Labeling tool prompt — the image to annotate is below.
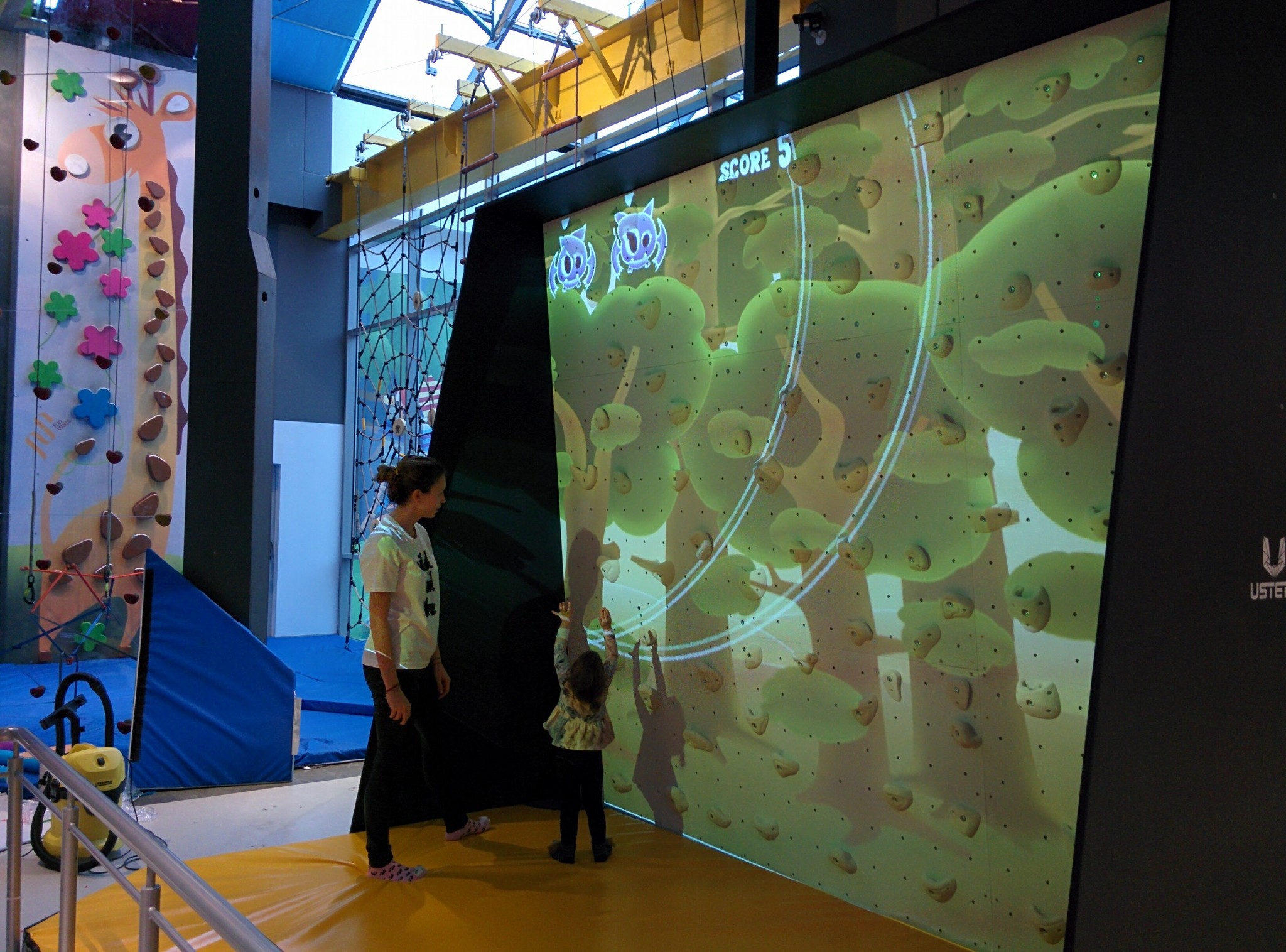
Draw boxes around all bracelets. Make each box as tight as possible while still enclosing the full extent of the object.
[385,682,400,693]
[433,662,442,665]
[432,657,441,662]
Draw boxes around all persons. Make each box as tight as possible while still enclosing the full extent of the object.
[355,454,499,883]
[541,601,620,869]
